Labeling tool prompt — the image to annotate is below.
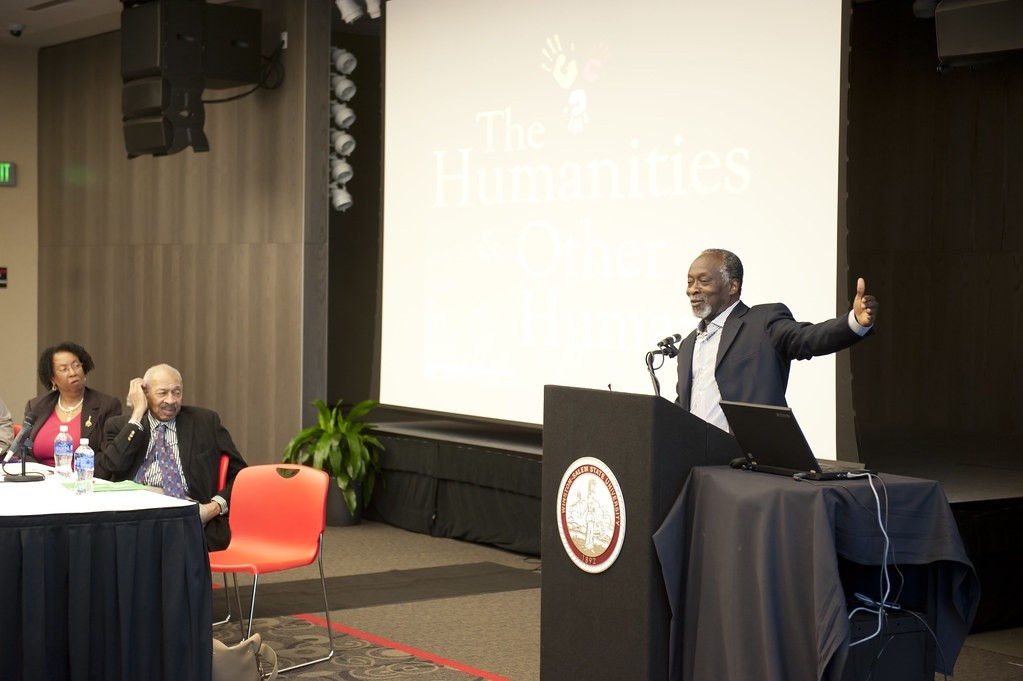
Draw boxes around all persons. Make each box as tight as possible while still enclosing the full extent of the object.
[24,341,122,481]
[675,249,878,437]
[0,401,14,457]
[100,364,246,553]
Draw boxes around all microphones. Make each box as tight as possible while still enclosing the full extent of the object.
[2,412,38,467]
[658,334,681,347]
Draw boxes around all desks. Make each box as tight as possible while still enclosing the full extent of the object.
[0,457,213,681]
[652,460,980,681]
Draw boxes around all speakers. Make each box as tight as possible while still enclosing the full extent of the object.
[935,0,1023,68]
[120,0,264,159]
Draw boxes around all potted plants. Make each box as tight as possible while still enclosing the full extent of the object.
[283,399,385,526]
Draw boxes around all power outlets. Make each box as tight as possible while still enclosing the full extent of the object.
[281,32,287,48]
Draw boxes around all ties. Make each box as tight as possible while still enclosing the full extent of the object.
[134,424,186,499]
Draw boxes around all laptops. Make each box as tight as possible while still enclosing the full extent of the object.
[719,400,878,480]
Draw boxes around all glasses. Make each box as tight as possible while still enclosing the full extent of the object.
[55,363,84,375]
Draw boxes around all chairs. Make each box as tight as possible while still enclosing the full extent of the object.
[208,463,333,677]
[213,453,230,626]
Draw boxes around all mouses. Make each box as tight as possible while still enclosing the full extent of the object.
[729,457,746,468]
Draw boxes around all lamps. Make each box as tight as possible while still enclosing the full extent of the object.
[331,46,357,74]
[330,100,356,128]
[329,183,352,211]
[335,0,363,24]
[365,0,381,19]
[330,73,356,100]
[329,127,355,156]
[329,155,353,183]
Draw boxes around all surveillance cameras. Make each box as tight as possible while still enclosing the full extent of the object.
[9,24,23,37]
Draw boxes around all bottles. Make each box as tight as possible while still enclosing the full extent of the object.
[74,438,95,496]
[54,425,74,483]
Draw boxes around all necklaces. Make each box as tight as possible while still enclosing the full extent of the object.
[58,394,83,413]
